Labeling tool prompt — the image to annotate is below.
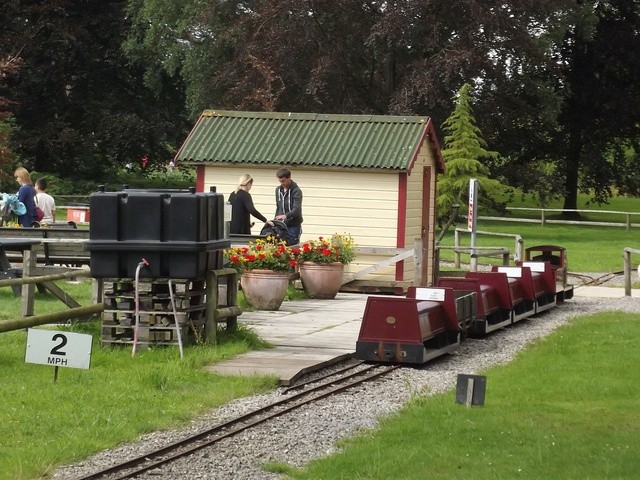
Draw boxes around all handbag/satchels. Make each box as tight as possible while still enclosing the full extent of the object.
[261,218,290,246]
[34,195,44,221]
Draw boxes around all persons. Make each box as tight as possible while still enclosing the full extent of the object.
[274,169,304,247]
[13,166,39,228]
[227,174,274,235]
[33,178,56,224]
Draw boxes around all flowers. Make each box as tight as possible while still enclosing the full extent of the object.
[298,233,360,266]
[229,234,301,273]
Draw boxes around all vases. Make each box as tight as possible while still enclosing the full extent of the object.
[298,260,344,299]
[240,268,288,310]
